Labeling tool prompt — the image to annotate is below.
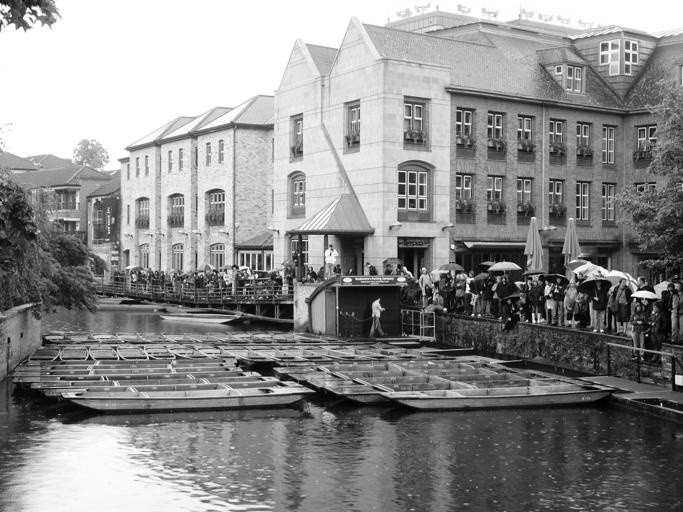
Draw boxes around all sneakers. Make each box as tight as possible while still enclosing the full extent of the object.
[440,307,629,345]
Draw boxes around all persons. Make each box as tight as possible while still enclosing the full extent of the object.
[364,262,378,275]
[367,296,389,339]
[293,249,305,278]
[384,264,395,275]
[111,262,354,301]
[323,244,338,280]
[401,263,682,363]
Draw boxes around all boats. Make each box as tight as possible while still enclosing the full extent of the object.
[91,299,168,312]
[152,308,246,325]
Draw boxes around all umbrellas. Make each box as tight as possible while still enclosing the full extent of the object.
[486,260,522,279]
[523,216,543,282]
[381,257,405,266]
[480,262,494,267]
[565,260,593,270]
[560,216,582,276]
